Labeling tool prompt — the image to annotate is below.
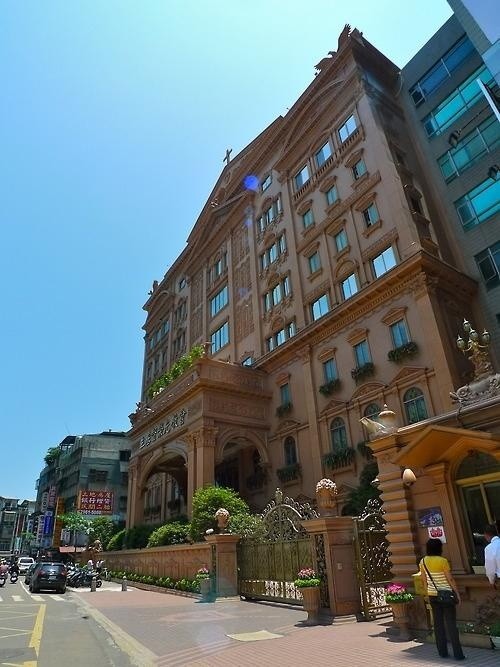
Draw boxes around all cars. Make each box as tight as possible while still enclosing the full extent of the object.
[23,563,34,584]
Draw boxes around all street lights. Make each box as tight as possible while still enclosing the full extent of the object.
[455,317,497,384]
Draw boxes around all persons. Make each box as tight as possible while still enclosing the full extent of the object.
[483,525,500,592]
[0,561,20,575]
[418,539,465,660]
[87,558,106,571]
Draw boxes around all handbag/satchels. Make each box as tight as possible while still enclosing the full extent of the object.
[437,590,457,605]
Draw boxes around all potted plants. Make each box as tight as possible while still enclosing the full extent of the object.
[490,623,500,649]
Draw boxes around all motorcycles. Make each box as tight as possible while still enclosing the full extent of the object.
[0,562,19,588]
[66,564,104,590]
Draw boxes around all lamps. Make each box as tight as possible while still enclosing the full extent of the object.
[402,467,417,487]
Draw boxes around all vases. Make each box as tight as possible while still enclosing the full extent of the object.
[198,577,210,602]
[297,585,320,626]
[388,600,414,640]
[460,633,493,649]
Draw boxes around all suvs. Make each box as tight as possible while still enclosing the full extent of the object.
[24,563,67,594]
[15,556,36,575]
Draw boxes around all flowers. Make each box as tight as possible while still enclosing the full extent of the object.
[293,567,320,587]
[196,567,210,578]
[384,584,414,603]
[463,622,490,634]
